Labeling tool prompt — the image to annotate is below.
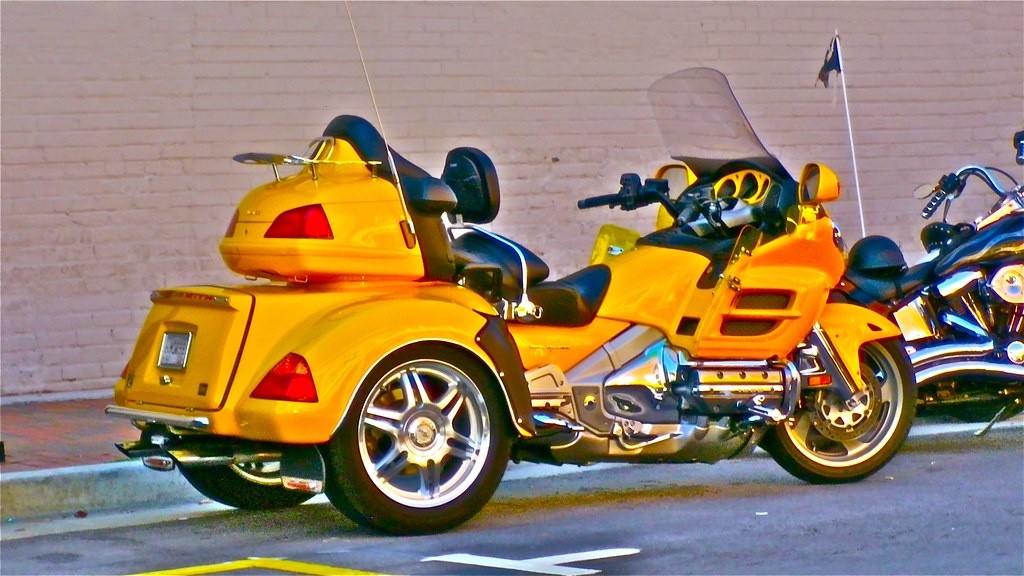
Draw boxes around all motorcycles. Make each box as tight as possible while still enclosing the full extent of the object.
[876,130,1024,438]
[98,68,916,539]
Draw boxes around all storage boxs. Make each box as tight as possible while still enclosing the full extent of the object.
[218,139,423,284]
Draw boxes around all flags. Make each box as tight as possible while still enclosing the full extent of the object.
[814,35,841,89]
